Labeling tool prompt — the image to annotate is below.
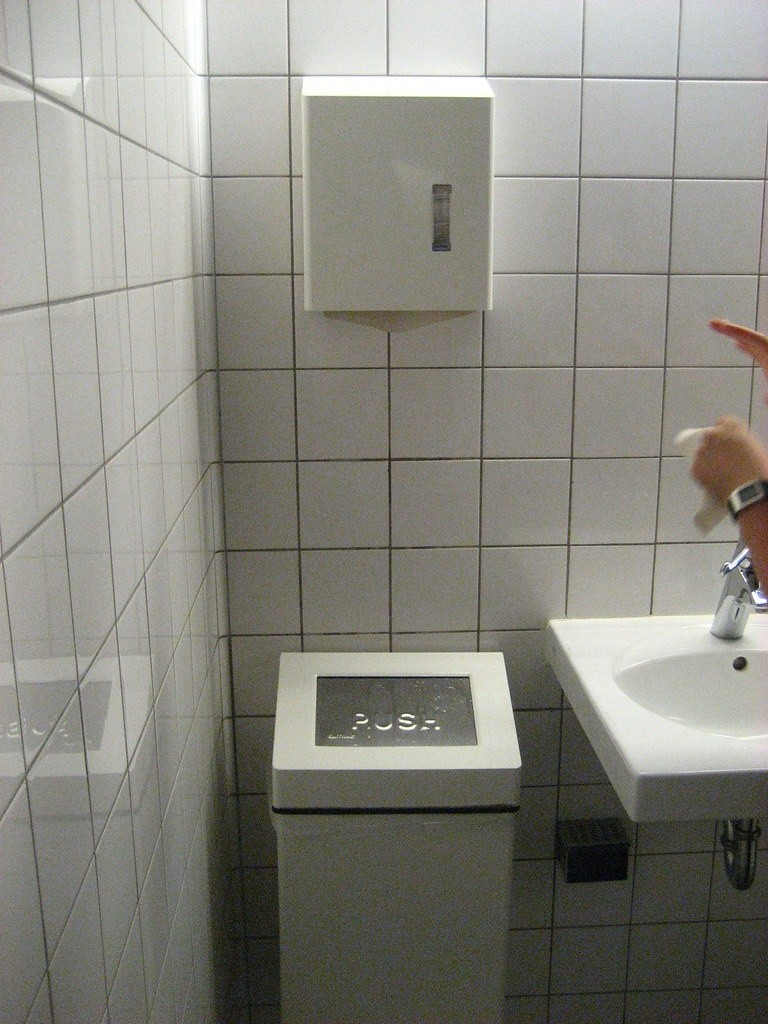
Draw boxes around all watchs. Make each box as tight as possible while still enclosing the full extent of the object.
[728,479,768,525]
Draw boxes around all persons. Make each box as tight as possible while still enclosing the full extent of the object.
[690,321,768,594]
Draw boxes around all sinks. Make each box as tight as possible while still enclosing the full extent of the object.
[542,609,768,823]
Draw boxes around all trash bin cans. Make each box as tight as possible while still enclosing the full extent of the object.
[267,651,524,1024]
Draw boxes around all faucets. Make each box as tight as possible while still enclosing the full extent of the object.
[708,535,768,641]
